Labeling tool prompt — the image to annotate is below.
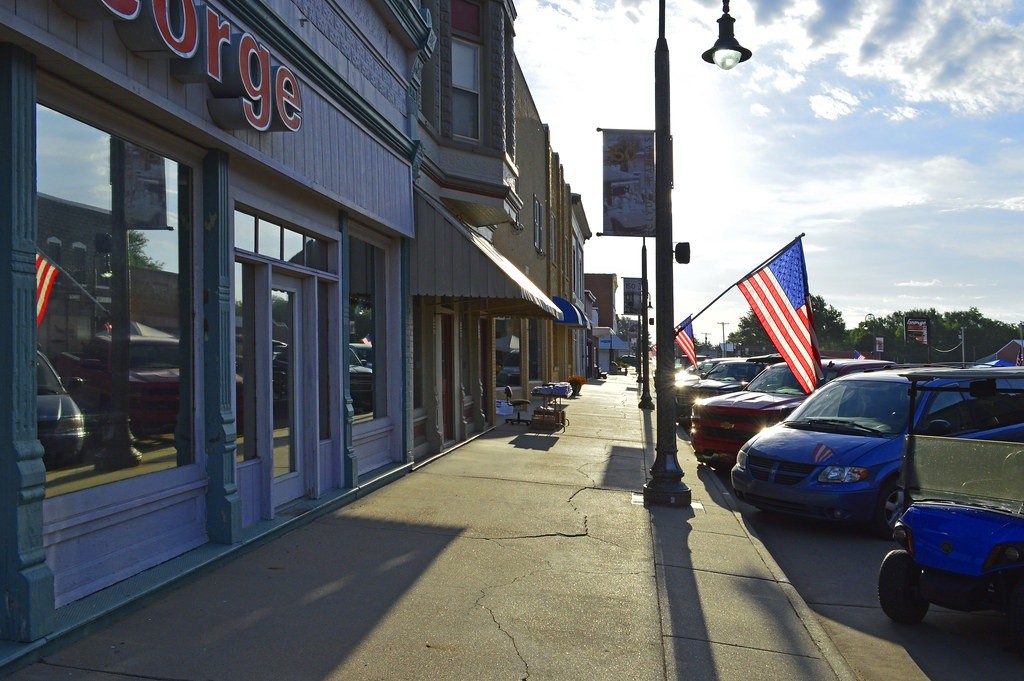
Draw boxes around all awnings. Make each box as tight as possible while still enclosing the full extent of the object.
[410,186,564,321]
[553,295,591,329]
[613,335,633,350]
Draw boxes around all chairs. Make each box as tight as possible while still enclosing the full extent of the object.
[504,385,531,425]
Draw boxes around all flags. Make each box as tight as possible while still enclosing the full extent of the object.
[35,253,60,328]
[736,235,824,394]
[853,349,866,359]
[813,442,834,463]
[1016,347,1022,366]
[674,314,698,369]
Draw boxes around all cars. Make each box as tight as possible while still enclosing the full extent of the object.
[878,363,1024,655]
[675,352,784,424]
[730,364,1024,540]
[689,357,911,468]
[498,348,538,381]
[93,332,246,437]
[35,343,92,468]
[237,334,372,408]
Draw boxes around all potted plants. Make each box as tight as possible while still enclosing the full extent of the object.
[567,375,587,396]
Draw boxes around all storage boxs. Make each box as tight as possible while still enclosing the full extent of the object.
[532,410,565,430]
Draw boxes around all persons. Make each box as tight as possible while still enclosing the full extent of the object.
[83,316,112,443]
[496,358,505,374]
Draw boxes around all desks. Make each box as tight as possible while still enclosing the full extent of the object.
[530,390,573,432]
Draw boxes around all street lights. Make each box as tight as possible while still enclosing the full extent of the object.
[634,290,654,384]
[861,313,877,360]
[700,332,712,355]
[717,322,729,358]
[643,0,753,509]
